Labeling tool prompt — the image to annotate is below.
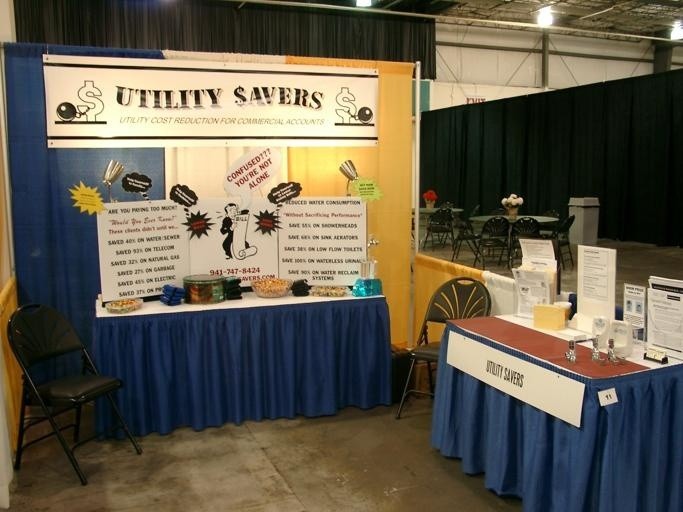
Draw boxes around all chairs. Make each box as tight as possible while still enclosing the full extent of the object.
[5,302,143,485]
[423,201,575,275]
[394,276,492,418]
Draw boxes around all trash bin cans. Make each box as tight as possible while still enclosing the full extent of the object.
[568,197,600,260]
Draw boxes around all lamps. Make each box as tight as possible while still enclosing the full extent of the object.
[338,159,358,195]
[103,160,125,204]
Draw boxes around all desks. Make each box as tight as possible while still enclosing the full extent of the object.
[433,312,676,512]
[95,288,390,438]
[419,207,465,243]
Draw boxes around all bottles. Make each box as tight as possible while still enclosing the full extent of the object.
[566,337,619,367]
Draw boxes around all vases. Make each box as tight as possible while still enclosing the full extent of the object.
[425,199,435,209]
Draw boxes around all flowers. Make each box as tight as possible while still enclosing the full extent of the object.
[422,190,437,200]
[502,193,524,208]
